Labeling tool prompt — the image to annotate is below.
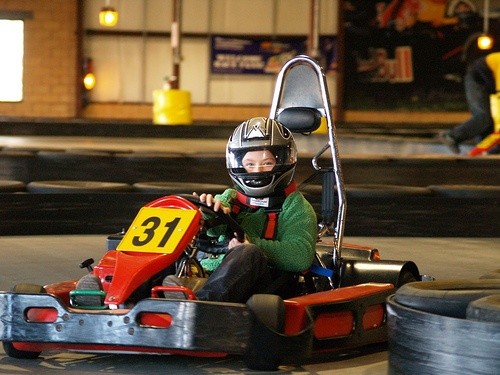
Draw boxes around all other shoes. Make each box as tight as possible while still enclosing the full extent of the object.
[76,275,103,306]
[162,275,187,300]
[433,129,462,153]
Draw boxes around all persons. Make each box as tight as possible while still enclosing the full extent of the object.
[440,52,500,154]
[73,116,319,302]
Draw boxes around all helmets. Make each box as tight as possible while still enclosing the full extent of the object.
[226,117,298,198]
